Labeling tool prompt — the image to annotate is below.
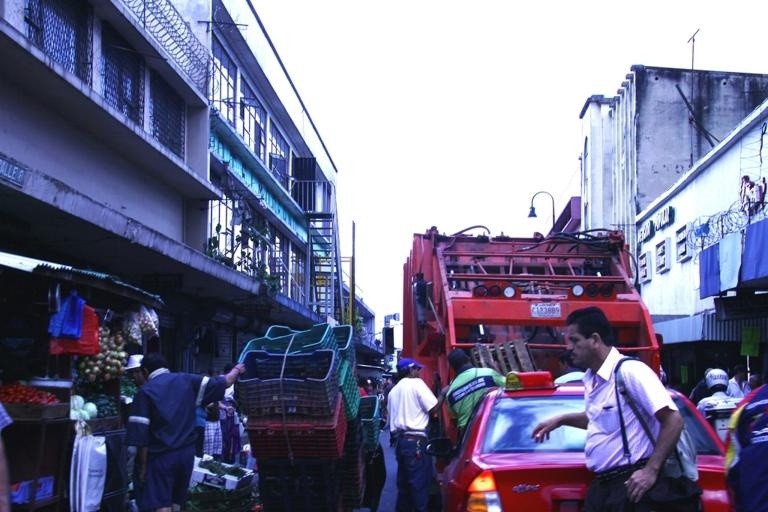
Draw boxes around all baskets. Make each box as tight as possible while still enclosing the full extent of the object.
[3,403,70,418]
[233,323,381,512]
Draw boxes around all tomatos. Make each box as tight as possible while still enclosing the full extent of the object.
[0,383,60,405]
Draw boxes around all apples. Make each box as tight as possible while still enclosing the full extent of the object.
[78,313,160,390]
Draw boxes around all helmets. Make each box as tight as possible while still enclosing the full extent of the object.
[706,369,728,389]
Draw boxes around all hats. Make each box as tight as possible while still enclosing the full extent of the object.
[448,348,464,362]
[396,358,425,371]
[123,354,145,370]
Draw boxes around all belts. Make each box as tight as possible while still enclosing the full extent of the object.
[594,458,649,480]
[398,434,422,440]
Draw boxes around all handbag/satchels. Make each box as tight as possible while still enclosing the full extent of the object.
[628,475,704,510]
[70,421,107,512]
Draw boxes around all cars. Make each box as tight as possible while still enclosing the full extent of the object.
[426,367,737,511]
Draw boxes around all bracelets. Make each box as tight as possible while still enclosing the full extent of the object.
[440,392,446,397]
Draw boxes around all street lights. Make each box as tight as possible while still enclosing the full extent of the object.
[528,191,557,235]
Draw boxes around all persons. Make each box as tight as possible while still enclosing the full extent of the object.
[121,394,134,482]
[354,371,399,447]
[554,351,586,384]
[529,305,684,511]
[121,353,147,387]
[722,382,767,512]
[388,358,454,512]
[124,350,246,512]
[193,362,245,462]
[0,402,14,512]
[446,348,506,441]
[687,365,761,417]
